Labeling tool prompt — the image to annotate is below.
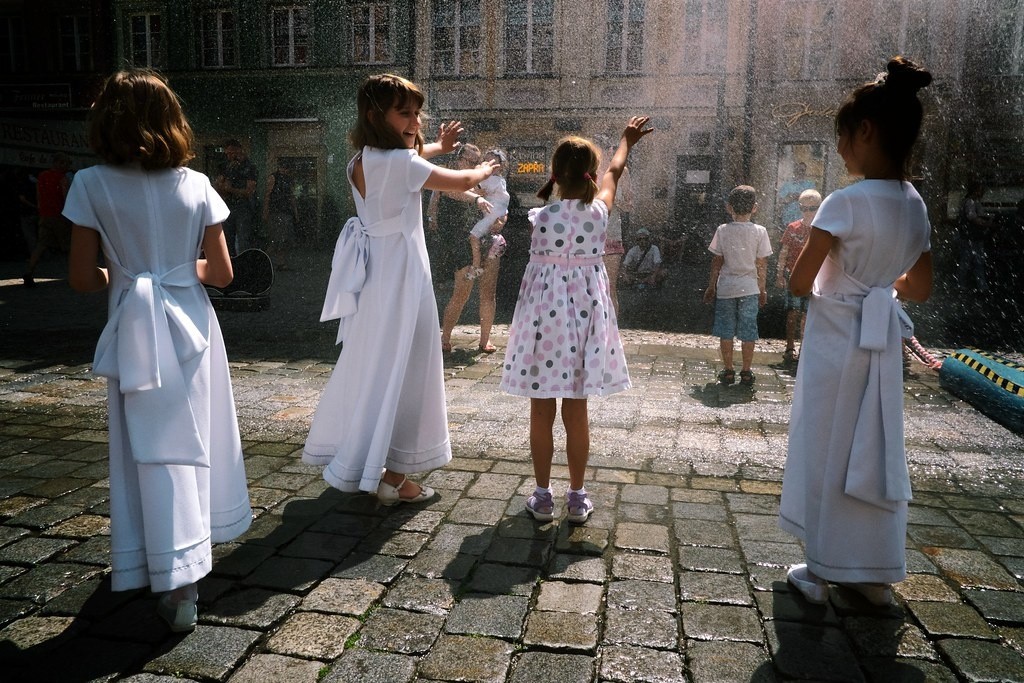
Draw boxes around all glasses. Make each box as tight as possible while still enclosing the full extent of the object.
[635,236,649,242]
[798,202,819,212]
[459,156,478,166]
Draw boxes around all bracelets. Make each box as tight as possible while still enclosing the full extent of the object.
[475,195,483,207]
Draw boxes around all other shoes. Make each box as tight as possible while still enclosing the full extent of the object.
[852,579,892,605]
[789,564,830,603]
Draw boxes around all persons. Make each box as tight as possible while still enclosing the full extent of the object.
[954,182,996,318]
[776,189,822,365]
[499,115,654,521]
[214,142,300,270]
[428,142,510,352]
[63,68,250,633]
[591,134,633,317]
[781,58,934,605]
[705,186,773,384]
[620,229,668,292]
[778,162,814,226]
[301,74,501,504]
[22,154,69,285]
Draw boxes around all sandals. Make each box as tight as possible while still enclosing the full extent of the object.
[526,493,555,520]
[441,342,452,352]
[157,597,198,632]
[376,476,435,507]
[716,368,736,384]
[463,265,487,283]
[567,492,594,523]
[739,369,756,386]
[782,349,799,362]
[480,340,497,352]
